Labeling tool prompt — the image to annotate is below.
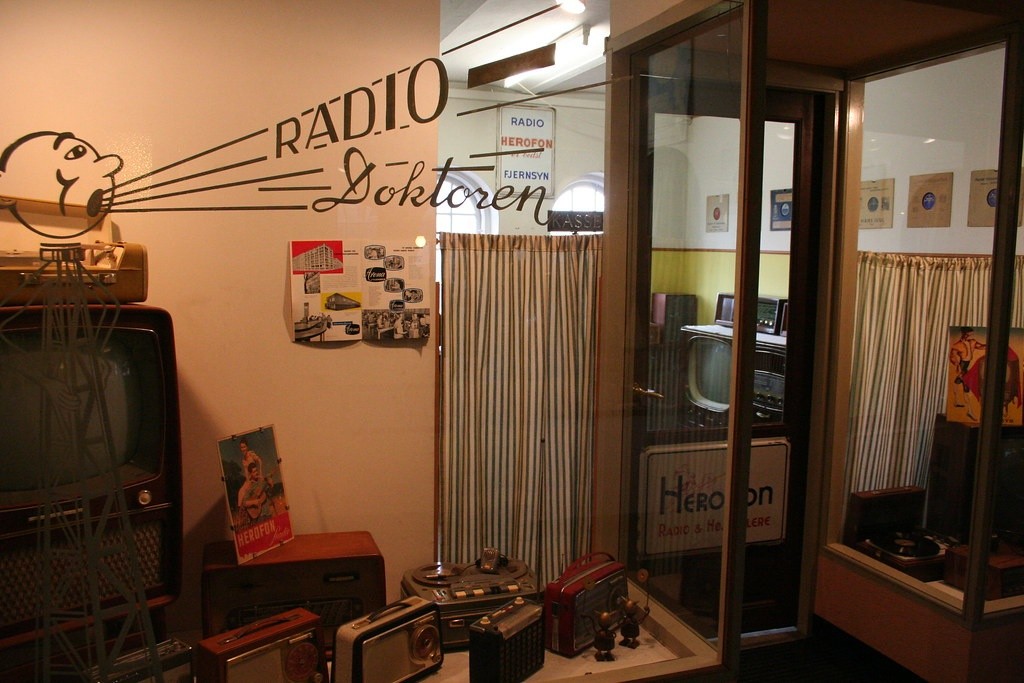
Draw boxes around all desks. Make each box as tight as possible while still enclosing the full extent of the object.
[816,542,1024,683]
[326,578,722,683]
[649,347,685,430]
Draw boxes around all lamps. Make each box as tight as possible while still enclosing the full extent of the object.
[556,0,586,14]
[502,23,590,87]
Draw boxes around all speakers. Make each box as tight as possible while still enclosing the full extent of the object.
[0,503,180,639]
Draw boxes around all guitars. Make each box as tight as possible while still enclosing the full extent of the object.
[244,472,275,521]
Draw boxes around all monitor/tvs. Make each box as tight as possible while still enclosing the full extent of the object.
[714,291,788,334]
[0,305,182,535]
[680,323,787,427]
[927,414,1024,547]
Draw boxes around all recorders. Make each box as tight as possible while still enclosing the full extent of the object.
[544,552,630,658]
[468,596,545,683]
[330,597,444,683]
[83,637,194,683]
[201,530,386,662]
[196,606,329,683]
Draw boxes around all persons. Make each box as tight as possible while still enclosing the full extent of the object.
[363,311,429,337]
[391,301,395,309]
[238,441,274,524]
[386,280,401,291]
[367,269,385,280]
[367,247,382,258]
[385,257,402,268]
[405,290,421,301]
